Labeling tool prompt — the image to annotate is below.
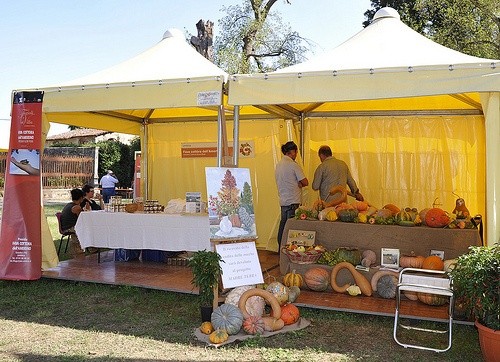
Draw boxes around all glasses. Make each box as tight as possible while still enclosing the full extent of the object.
[89,190,94,193]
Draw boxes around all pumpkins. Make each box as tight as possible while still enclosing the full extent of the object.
[281,184,471,306]
[200,281,299,344]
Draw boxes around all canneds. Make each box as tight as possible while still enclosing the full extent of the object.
[107,196,127,212]
[143,200,162,211]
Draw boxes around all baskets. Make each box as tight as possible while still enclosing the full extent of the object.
[282,247,322,264]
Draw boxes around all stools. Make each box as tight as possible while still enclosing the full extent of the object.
[393,268,454,353]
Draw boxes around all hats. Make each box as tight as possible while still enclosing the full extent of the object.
[108,170,113,174]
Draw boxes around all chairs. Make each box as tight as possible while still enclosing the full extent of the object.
[55,212,76,255]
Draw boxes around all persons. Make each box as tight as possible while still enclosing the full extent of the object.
[312,146,364,203]
[60,185,104,253]
[10,157,39,175]
[100,171,118,203]
[275,141,309,251]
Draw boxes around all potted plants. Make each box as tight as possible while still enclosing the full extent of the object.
[186,249,226,323]
[444,243,500,362]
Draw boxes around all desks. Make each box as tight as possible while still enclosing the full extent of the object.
[91,188,133,201]
[74,210,214,263]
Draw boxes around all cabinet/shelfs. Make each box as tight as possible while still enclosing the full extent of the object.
[280,217,482,295]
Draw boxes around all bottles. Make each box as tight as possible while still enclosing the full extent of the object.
[86,202,90,211]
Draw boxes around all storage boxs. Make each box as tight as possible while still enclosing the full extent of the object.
[113,247,169,264]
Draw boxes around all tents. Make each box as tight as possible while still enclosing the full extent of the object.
[229,8,500,245]
[11,30,300,253]
